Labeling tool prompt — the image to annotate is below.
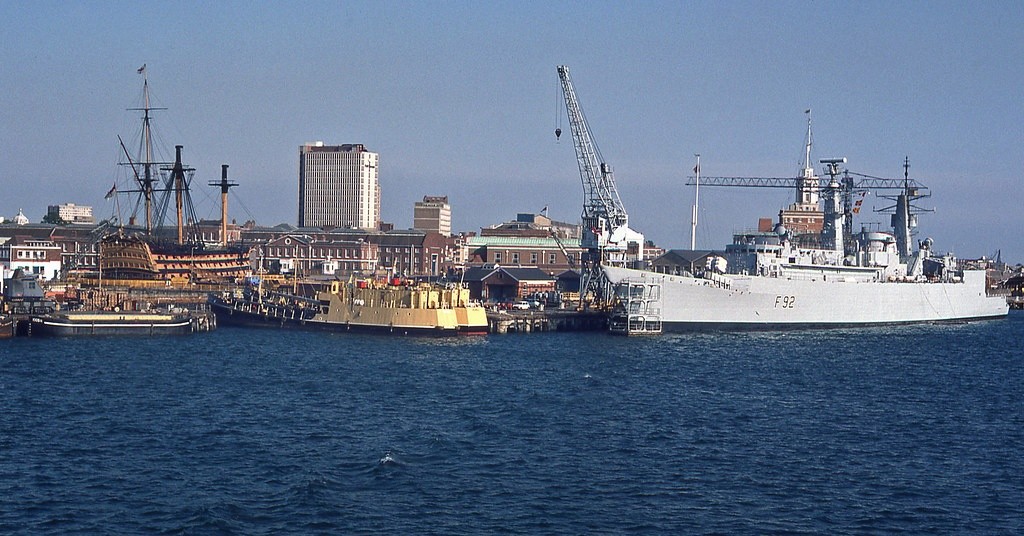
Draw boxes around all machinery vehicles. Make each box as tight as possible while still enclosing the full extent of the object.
[554,64,631,251]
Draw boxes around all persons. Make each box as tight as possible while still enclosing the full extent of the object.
[171,306,189,314]
[56,302,60,311]
[145,301,157,313]
[534,289,548,307]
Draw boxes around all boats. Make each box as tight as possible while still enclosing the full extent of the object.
[211,268,490,337]
[26,308,193,335]
[600,155,1011,334]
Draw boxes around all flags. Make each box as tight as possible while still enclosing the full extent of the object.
[853,192,866,213]
[541,206,547,212]
